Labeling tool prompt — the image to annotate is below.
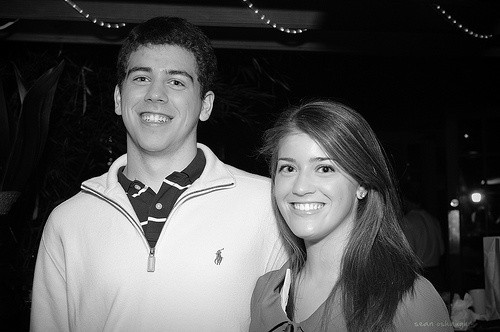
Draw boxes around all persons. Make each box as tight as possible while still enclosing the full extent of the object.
[398,182,446,289]
[248,100,458,332]
[31,16,295,332]
